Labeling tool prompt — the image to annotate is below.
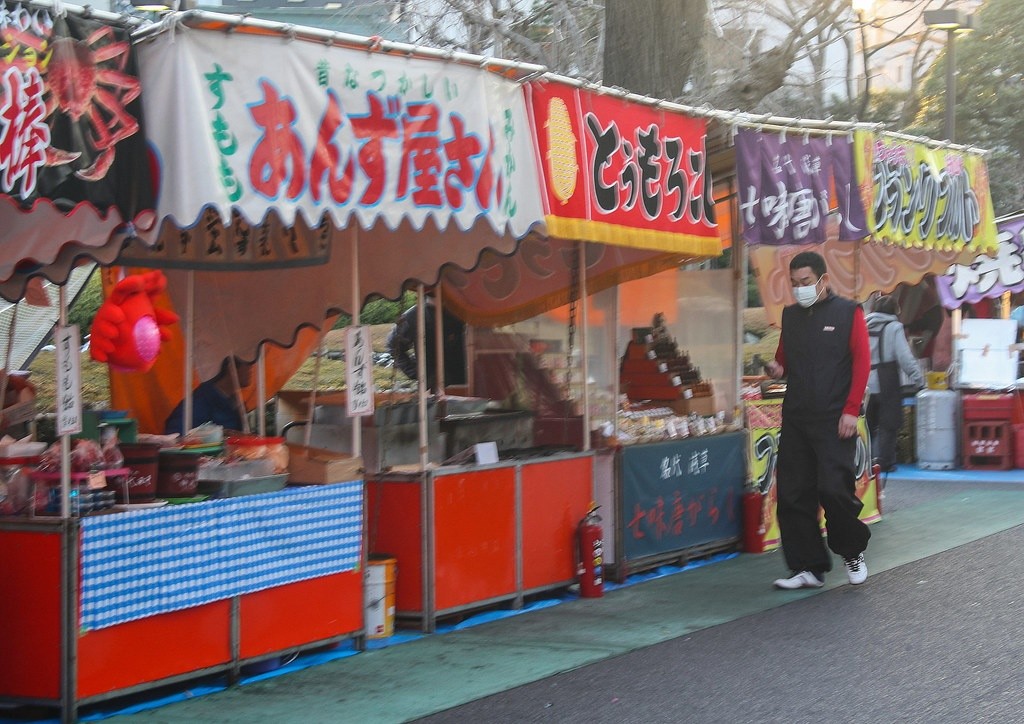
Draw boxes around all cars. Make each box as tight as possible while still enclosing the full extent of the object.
[320,350,345,360]
[311,352,319,356]
[372,351,394,365]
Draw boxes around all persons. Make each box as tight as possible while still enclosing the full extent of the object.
[904,298,975,372]
[865,294,926,472]
[165,358,252,435]
[765,252,871,590]
[0,368,37,438]
[386,297,466,395]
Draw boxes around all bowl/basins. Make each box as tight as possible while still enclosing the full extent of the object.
[197,461,292,499]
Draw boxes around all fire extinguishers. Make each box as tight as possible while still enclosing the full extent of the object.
[743,480,764,553]
[574,504,604,598]
[872,457,887,514]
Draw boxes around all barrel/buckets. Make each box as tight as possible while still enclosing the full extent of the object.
[119,443,160,504]
[159,454,198,498]
[365,551,398,642]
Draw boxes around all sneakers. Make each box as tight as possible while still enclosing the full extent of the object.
[842,551,867,584]
[772,571,823,589]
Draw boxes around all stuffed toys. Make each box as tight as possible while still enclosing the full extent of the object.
[91,270,182,372]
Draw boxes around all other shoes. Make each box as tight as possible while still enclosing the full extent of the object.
[880,465,899,472]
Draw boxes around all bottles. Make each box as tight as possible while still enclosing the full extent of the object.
[227,434,288,474]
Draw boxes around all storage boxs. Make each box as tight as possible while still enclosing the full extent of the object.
[286,444,360,484]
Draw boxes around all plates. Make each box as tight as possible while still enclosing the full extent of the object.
[98,410,129,419]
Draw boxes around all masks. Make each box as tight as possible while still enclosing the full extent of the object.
[792,274,824,308]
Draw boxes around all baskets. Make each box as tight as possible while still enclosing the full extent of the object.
[288,443,360,485]
